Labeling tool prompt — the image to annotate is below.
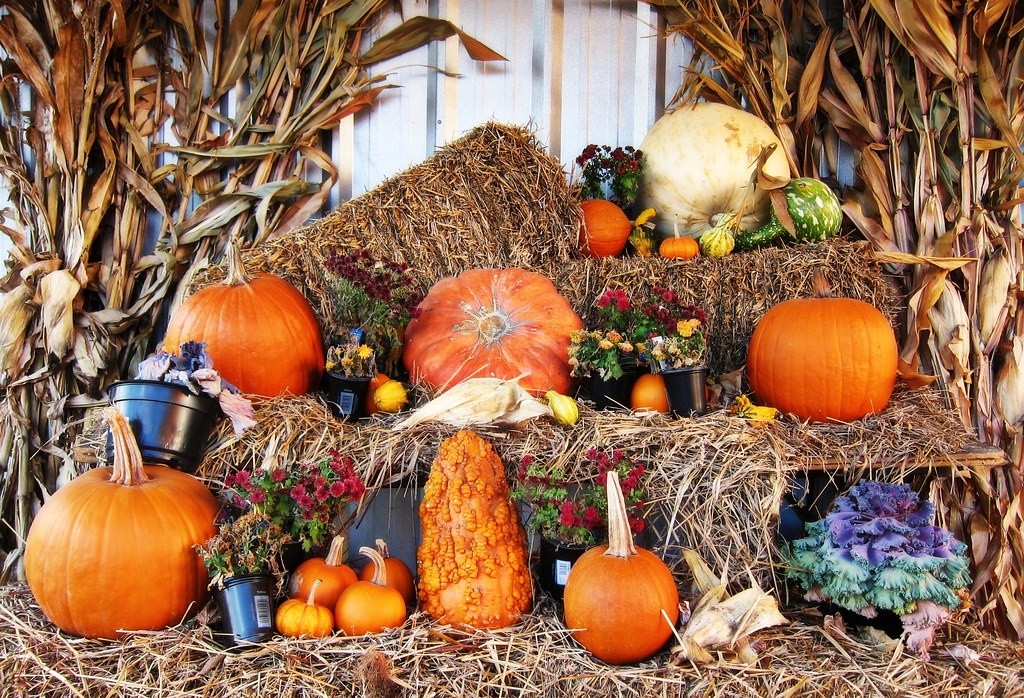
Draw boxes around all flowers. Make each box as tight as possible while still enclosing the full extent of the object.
[191,450,365,591]
[509,448,646,546]
[320,250,426,378]
[779,477,974,659]
[576,143,645,205]
[133,339,256,435]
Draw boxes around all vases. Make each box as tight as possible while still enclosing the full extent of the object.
[659,366,710,417]
[567,286,711,382]
[621,203,642,221]
[208,570,275,653]
[581,370,615,407]
[616,366,650,408]
[104,379,217,472]
[830,603,904,640]
[326,371,372,422]
[539,532,604,601]
[276,541,327,586]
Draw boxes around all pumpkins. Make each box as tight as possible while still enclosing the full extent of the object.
[165,236,323,405]
[276,535,411,637]
[578,103,843,258]
[563,471,678,663]
[631,375,669,413]
[405,268,586,397]
[24,417,226,639]
[746,266,898,425]
[368,374,408,413]
[417,433,535,632]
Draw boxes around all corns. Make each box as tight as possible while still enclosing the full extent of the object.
[935,249,1024,426]
